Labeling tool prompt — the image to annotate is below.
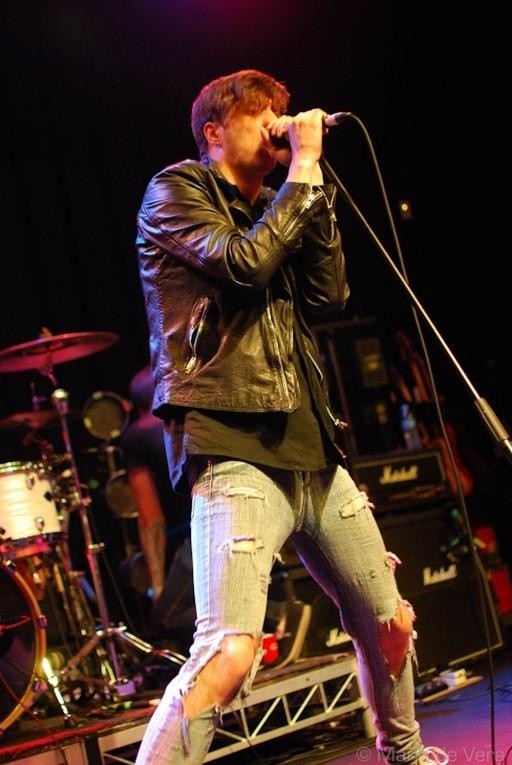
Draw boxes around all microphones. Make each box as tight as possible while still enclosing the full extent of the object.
[269,111,352,149]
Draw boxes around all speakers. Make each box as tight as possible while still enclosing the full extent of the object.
[382,505,500,684]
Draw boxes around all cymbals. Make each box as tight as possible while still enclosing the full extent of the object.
[0,331,119,375]
[2,411,73,435]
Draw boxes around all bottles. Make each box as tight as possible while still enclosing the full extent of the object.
[399,403,423,452]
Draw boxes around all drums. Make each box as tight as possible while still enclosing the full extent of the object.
[0,460,65,547]
[0,553,49,741]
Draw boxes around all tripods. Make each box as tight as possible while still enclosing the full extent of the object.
[40,360,187,697]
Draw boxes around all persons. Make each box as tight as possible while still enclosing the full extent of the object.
[114,359,193,630]
[414,418,510,575]
[20,430,59,461]
[133,65,443,765]
